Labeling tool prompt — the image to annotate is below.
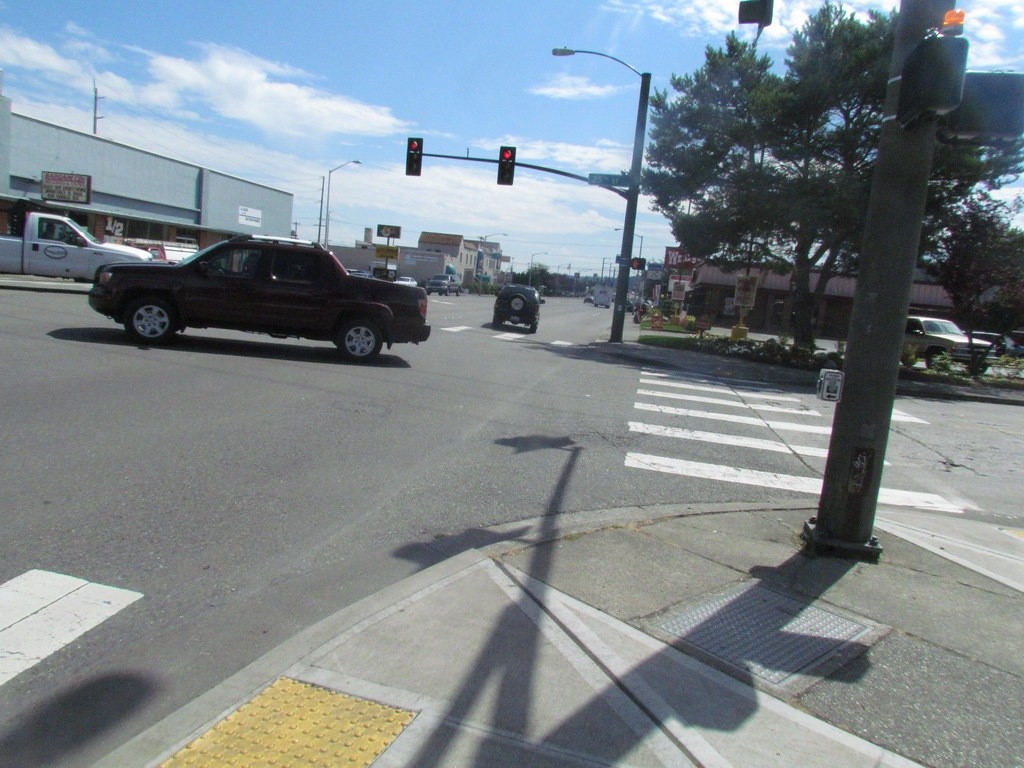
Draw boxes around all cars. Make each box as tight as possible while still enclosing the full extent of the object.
[584,296,594,304]
[346,269,373,278]
[394,276,418,287]
[962,331,1024,358]
[902,316,997,376]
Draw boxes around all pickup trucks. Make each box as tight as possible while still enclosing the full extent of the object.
[0,211,152,281]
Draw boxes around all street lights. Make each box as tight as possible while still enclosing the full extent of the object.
[528,252,548,286]
[614,228,644,315]
[552,45,651,345]
[324,160,362,250]
[480,234,509,295]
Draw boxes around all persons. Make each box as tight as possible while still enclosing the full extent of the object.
[41,222,56,239]
[996,336,1007,357]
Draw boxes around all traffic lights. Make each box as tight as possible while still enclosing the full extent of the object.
[406,137,423,176]
[497,146,517,186]
[631,258,645,270]
[895,37,968,130]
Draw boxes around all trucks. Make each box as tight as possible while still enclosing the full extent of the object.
[127,243,198,263]
[594,288,613,309]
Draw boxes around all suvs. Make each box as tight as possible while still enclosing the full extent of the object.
[88,235,432,364]
[492,284,546,334]
[425,274,463,296]
